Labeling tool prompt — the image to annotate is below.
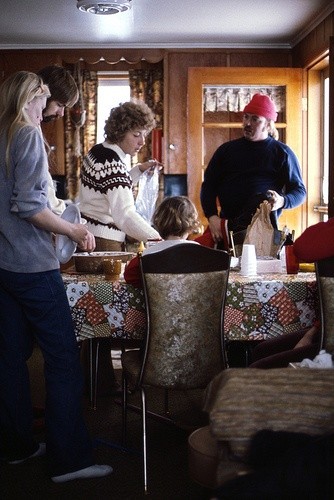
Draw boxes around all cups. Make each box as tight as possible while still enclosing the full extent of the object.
[286,244,300,274]
[239,244,257,276]
[102,261,121,280]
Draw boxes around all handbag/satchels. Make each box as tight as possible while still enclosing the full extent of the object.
[134,166,159,226]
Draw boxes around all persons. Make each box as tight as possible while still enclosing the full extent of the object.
[124,196,202,358]
[36,64,88,242]
[0,72,113,480]
[248,218,334,369]
[200,94,307,251]
[72,100,161,397]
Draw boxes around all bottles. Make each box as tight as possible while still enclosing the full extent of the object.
[137,240,145,256]
[279,245,288,272]
[277,225,295,259]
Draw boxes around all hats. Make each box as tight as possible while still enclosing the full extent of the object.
[243,94,277,121]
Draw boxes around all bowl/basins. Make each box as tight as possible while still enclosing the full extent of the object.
[300,261,316,272]
[70,250,137,274]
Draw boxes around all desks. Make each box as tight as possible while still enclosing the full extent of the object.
[61,263,318,413]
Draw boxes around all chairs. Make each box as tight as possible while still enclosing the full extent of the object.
[122,243,228,495]
[316,256,334,355]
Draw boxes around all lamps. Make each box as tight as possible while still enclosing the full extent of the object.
[76,0,132,16]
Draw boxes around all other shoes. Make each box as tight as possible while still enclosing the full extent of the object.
[117,388,132,395]
